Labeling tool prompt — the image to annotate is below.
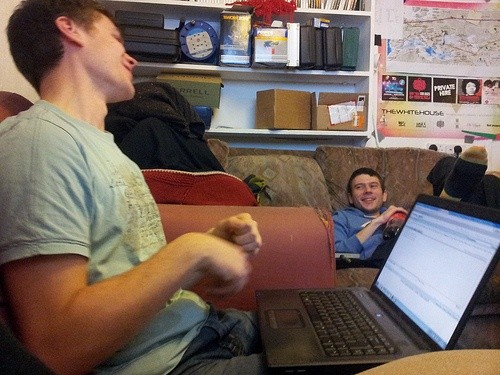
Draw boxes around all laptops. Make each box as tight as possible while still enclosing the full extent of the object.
[255,193,500,375]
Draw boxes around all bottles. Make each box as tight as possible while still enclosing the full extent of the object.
[383,211,406,241]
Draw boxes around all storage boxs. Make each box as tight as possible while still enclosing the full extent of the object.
[156,73,224,109]
[256,88,311,129]
[311,92,369,131]
[192,106,214,130]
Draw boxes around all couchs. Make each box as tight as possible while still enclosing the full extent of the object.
[206,139,500,316]
[0,90,336,375]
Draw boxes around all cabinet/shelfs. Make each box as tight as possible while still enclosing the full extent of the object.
[106,0,375,136]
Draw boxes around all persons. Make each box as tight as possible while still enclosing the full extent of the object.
[482,80,500,105]
[459,79,482,103]
[332,146,500,269]
[0,0,267,375]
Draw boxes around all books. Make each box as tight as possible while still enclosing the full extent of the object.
[296,0,366,11]
